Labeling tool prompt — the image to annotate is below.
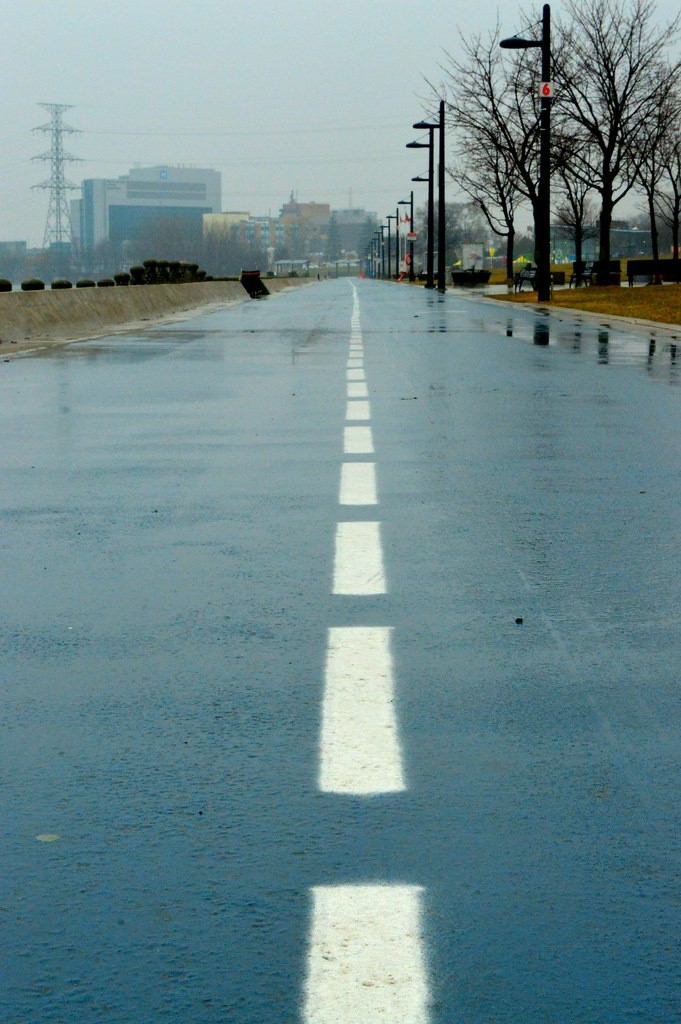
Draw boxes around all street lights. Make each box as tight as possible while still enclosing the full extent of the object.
[411,100,446,295]
[360,224,386,280]
[500,4,551,303]
[406,128,435,290]
[397,190,416,282]
[380,218,392,279]
[386,207,400,279]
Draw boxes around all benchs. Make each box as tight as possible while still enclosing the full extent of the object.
[511,264,622,293]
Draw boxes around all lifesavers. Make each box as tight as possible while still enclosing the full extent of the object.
[405,253,411,265]
